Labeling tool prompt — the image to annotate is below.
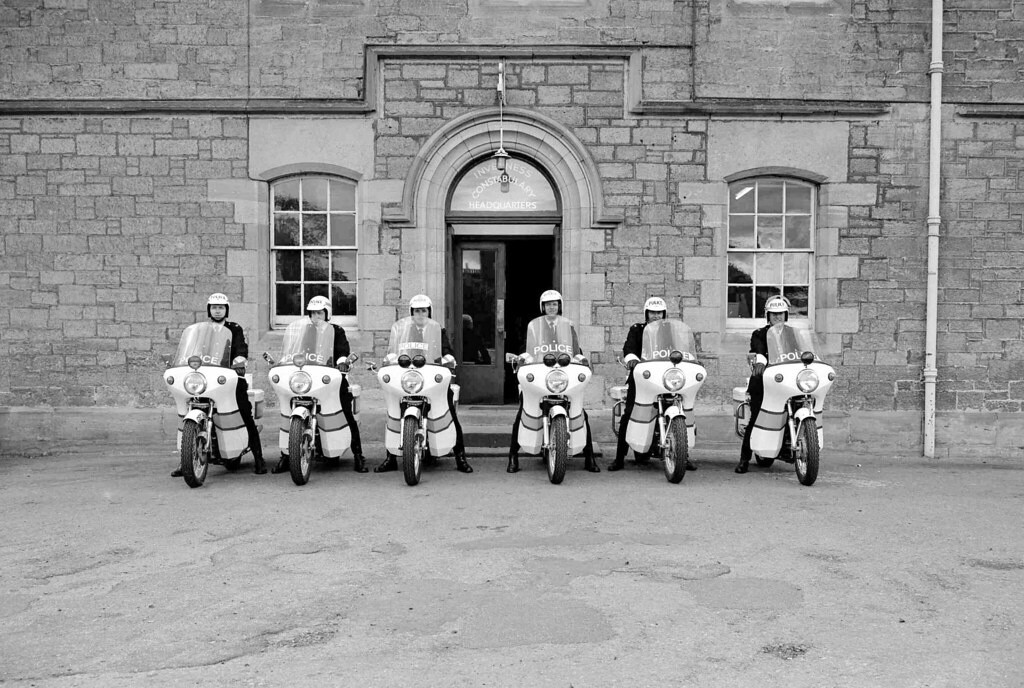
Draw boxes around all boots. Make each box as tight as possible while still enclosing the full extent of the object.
[353,454,369,474]
[455,451,473,474]
[373,454,399,473]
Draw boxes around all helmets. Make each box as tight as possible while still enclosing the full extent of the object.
[409,294,433,320]
[540,289,563,315]
[206,293,229,317]
[306,295,332,322]
[644,297,668,320]
[765,298,790,323]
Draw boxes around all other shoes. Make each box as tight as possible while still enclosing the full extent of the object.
[171,462,182,477]
[735,460,749,474]
[606,459,625,472]
[272,453,289,475]
[685,459,697,472]
[254,460,269,475]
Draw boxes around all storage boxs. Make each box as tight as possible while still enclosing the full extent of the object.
[732,387,752,425]
[610,386,627,430]
[450,384,461,412]
[247,389,265,425]
[349,384,361,420]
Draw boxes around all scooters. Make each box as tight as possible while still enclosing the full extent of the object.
[507,314,594,486]
[607,319,709,484]
[363,315,462,486]
[731,320,839,486]
[262,314,364,487]
[159,321,266,490]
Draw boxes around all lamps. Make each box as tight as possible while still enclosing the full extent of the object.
[491,62,511,170]
[496,158,515,193]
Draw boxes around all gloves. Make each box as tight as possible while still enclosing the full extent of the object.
[626,360,638,370]
[236,367,245,377]
[753,363,765,376]
[335,363,349,372]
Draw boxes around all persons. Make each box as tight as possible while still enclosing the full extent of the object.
[272,295,365,472]
[170,292,267,474]
[507,291,600,472]
[374,295,473,472]
[463,315,492,364]
[735,299,817,473]
[608,297,697,471]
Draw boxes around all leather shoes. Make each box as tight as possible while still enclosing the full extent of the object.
[506,455,520,473]
[584,457,601,474]
